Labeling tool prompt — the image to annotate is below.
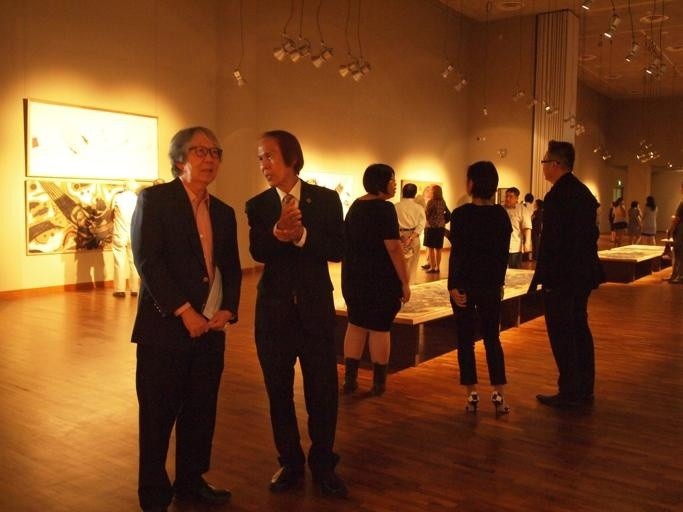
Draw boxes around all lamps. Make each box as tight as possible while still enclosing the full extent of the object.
[511,0,585,137]
[441,1,456,78]
[594,0,673,169]
[454,0,469,93]
[272,0,371,82]
[233,1,248,87]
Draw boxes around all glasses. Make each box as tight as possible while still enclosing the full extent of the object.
[187,145,224,158]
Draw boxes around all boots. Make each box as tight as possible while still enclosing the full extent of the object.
[372,361,387,395]
[344,358,359,391]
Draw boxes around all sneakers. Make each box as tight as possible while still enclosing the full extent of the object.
[114,290,137,298]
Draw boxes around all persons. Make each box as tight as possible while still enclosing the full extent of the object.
[395,183,451,277]
[523,140,600,412]
[111,180,139,298]
[131,128,242,512]
[609,196,683,284]
[500,187,544,268]
[341,164,411,397]
[245,131,349,498]
[447,162,513,416]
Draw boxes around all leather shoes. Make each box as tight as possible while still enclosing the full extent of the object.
[422,261,441,274]
[268,464,305,492]
[171,479,232,501]
[537,390,594,408]
[313,465,348,497]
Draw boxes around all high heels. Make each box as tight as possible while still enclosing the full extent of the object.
[466,391,479,417]
[492,393,511,416]
